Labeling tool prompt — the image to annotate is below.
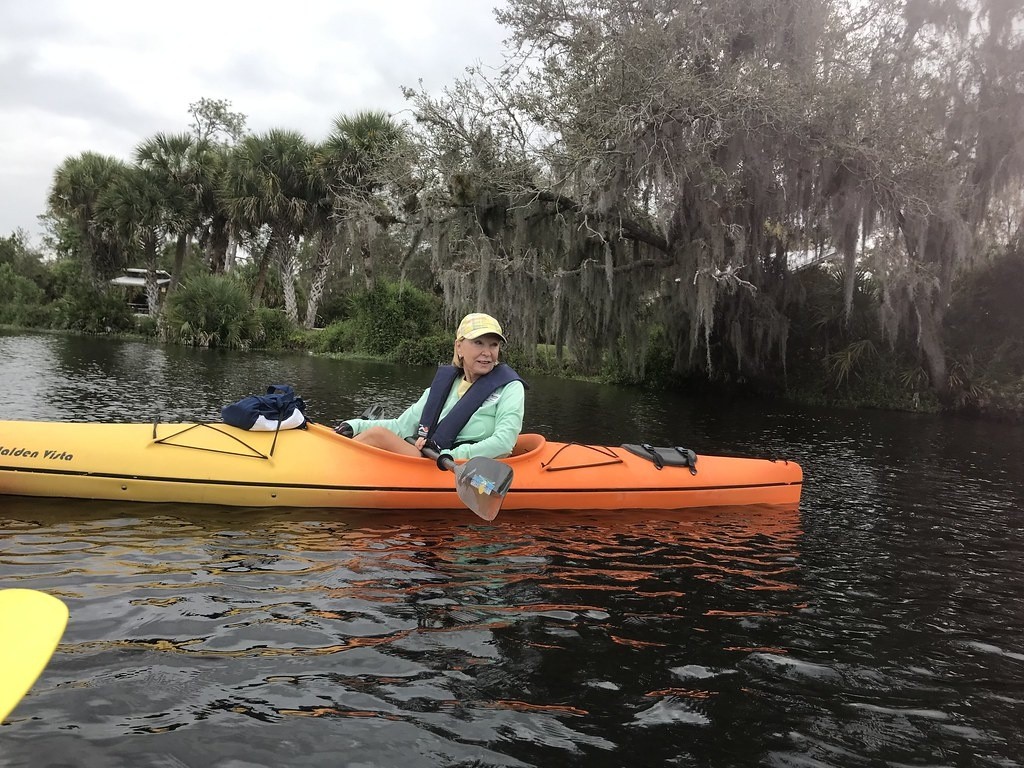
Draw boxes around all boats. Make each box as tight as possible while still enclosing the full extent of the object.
[0,391,803,510]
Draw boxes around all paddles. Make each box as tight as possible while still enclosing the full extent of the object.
[359,403,514,524]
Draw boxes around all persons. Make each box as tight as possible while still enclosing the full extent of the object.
[335,312,529,460]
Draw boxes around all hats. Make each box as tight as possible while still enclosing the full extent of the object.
[457,313,507,344]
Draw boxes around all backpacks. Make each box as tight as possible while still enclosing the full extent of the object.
[222,385,307,431]
